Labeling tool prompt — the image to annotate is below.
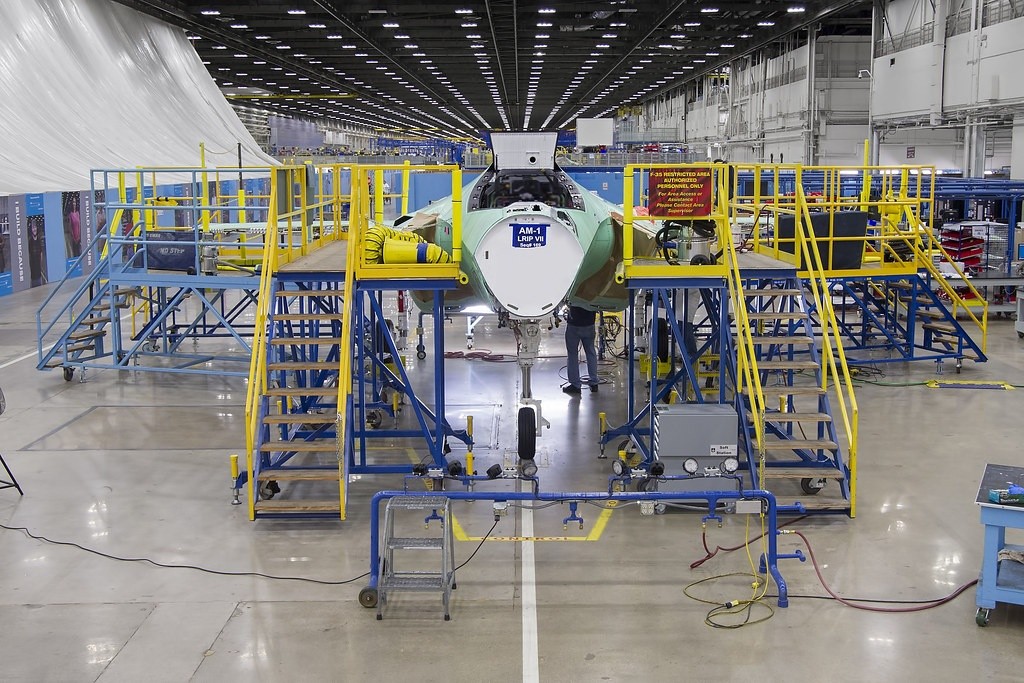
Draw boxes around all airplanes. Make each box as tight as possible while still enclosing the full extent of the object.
[194,129,775,458]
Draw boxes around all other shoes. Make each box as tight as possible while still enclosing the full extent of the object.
[563,384,581,393]
[590,385,598,392]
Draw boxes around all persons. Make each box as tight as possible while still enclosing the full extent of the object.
[68,196,82,257]
[28,220,43,289]
[561,307,599,395]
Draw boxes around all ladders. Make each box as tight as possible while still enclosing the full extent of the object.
[375,496,458,621]
